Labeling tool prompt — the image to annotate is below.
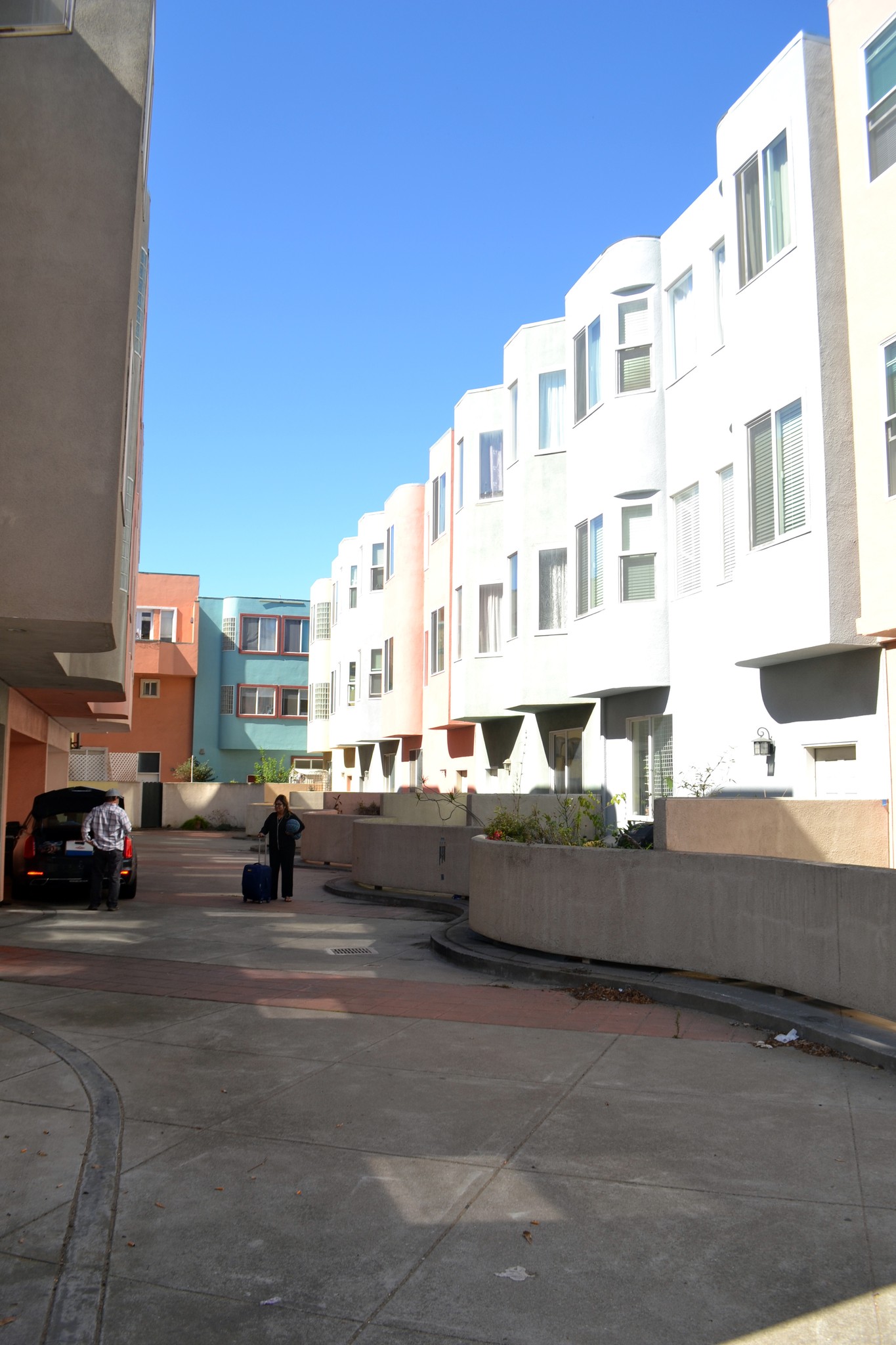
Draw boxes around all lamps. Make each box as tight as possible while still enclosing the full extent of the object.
[503,759,511,772]
[364,770,369,777]
[341,771,345,777]
[199,749,206,754]
[751,727,776,757]
[171,768,175,771]
[261,757,266,761]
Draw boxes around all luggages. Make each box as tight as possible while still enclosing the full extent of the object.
[242,835,273,904]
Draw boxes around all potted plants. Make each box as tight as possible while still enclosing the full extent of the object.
[181,814,213,830]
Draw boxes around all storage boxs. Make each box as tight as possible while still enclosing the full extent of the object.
[66,840,94,858]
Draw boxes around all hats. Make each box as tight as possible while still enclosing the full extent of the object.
[105,788,123,799]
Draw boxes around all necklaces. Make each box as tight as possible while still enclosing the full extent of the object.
[278,816,282,820]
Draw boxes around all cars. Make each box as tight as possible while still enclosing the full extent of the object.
[6,785,138,903]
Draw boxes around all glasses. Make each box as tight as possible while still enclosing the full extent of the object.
[274,802,283,806]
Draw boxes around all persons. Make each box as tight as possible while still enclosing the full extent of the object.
[258,794,305,901]
[81,789,132,910]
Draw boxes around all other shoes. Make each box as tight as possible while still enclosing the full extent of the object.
[285,897,292,902]
[88,905,98,910]
[108,906,119,911]
